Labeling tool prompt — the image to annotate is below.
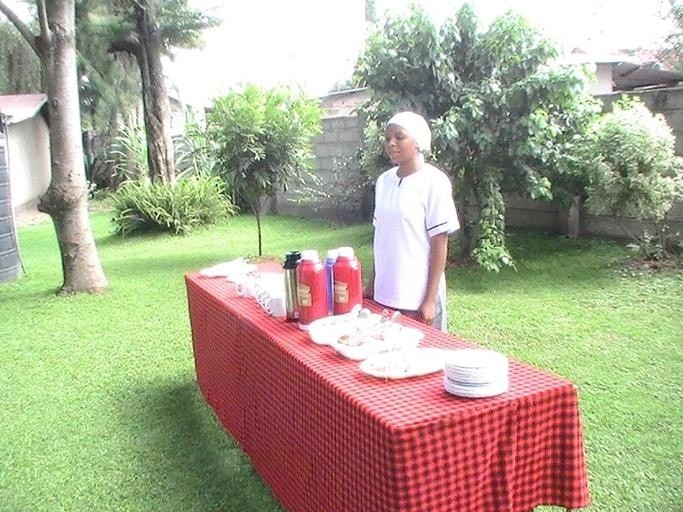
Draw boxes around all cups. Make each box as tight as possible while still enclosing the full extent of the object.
[235,270,286,318]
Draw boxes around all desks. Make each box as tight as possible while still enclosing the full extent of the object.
[184,256,591,512]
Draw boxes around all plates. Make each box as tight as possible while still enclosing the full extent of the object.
[308,311,381,345]
[442,347,509,399]
[359,347,451,380]
[334,327,421,361]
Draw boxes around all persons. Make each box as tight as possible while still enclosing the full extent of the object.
[362,111,461,332]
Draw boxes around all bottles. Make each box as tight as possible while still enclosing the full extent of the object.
[281,250,302,322]
[323,249,338,316]
[332,246,363,315]
[296,248,328,330]
